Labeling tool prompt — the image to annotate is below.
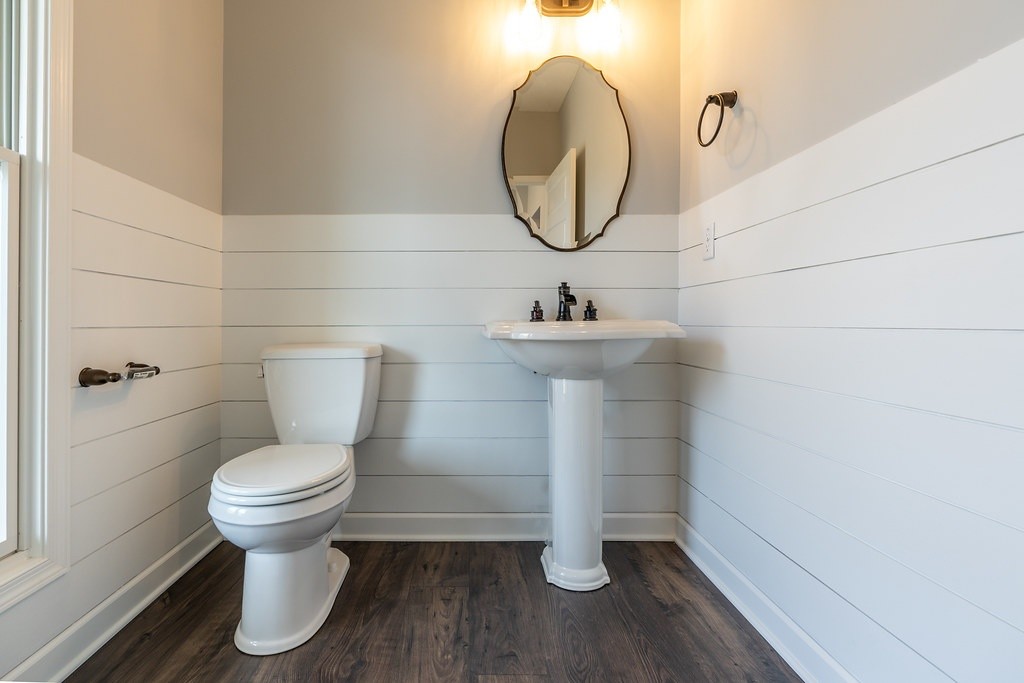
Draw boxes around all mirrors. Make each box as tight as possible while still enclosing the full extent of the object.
[501,55,632,252]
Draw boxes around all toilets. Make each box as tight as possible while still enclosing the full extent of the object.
[207,343,383,655]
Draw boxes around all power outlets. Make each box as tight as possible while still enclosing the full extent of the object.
[702,222,716,261]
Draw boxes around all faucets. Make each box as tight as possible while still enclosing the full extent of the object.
[556,282,578,322]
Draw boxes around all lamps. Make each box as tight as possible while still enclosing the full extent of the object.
[535,0,606,17]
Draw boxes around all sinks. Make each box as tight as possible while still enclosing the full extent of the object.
[483,319,687,380]
